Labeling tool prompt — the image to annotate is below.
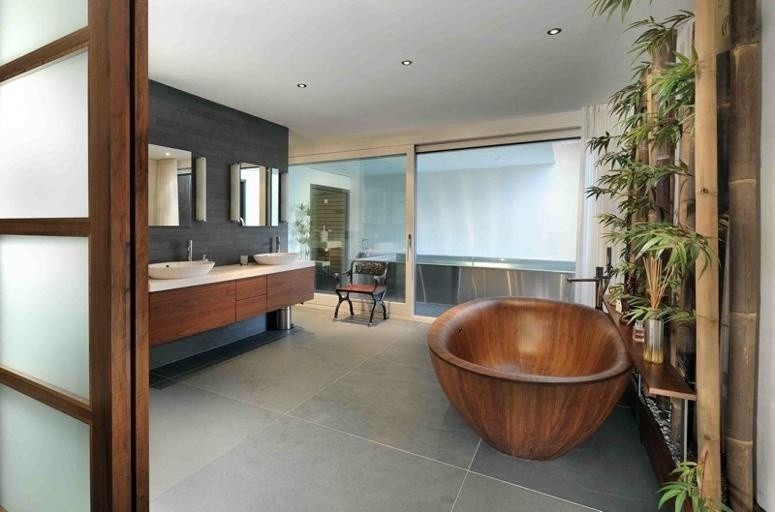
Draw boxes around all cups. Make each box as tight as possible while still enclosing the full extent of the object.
[240,255,249,266]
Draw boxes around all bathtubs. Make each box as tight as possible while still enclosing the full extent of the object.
[426,296,633,458]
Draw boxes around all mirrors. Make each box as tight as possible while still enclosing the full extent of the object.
[147,144,192,228]
[240,160,279,227]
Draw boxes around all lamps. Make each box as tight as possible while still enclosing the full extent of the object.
[195,155,209,223]
[280,171,290,223]
[231,160,240,223]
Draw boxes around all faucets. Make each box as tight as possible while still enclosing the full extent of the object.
[568,266,611,309]
[186,239,193,261]
[271,236,281,253]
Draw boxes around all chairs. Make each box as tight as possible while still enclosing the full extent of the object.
[315,248,331,281]
[332,259,390,326]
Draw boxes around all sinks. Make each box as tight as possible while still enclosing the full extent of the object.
[252,252,300,265]
[147,260,215,279]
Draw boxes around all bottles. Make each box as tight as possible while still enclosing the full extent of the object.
[632,318,646,344]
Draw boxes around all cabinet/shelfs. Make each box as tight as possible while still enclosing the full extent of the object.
[148,260,316,347]
[601,293,697,468]
